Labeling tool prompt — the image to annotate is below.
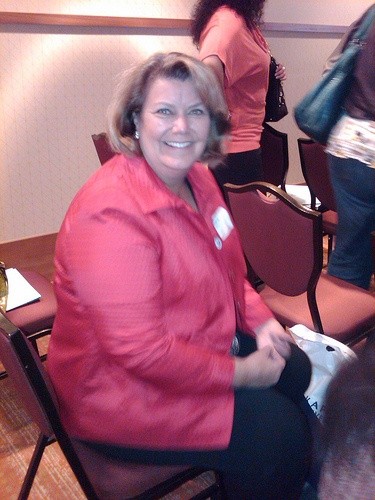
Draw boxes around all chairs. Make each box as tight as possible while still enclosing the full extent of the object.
[0,123,375,500]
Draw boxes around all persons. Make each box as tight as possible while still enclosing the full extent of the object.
[45,51,327,500]
[188,0,288,186]
[320,1,375,291]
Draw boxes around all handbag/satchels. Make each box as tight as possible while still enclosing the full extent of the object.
[266,56,289,123]
[286,322,358,427]
[294,8,375,146]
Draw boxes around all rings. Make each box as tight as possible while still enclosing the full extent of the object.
[283,66,286,70]
